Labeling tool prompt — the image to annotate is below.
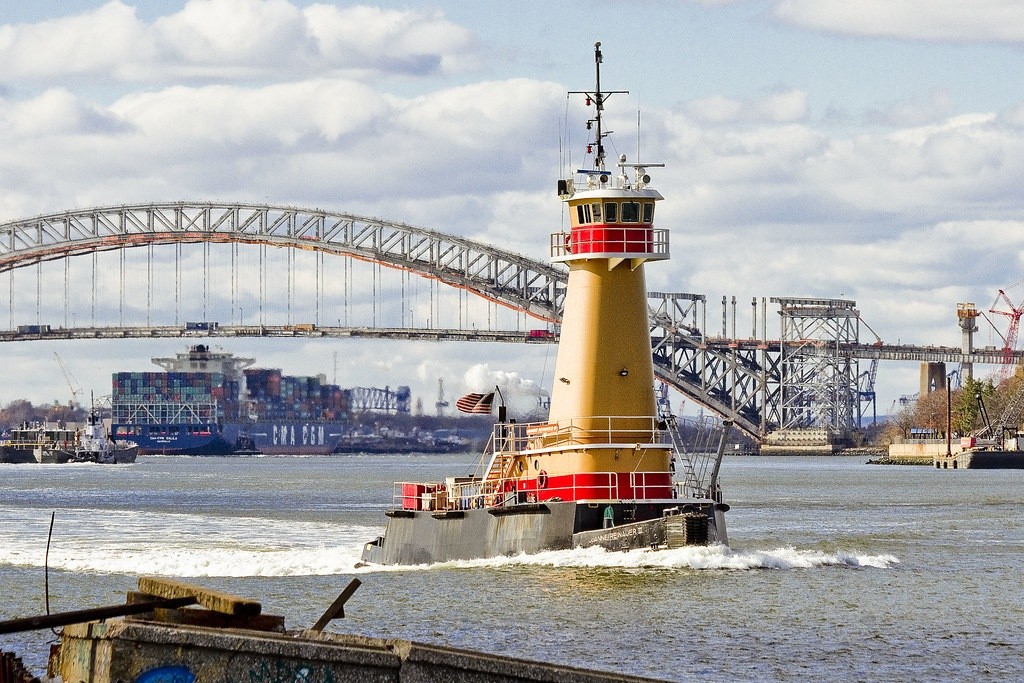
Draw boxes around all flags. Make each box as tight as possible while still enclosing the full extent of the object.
[456,393,495,415]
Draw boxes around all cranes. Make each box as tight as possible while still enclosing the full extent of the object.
[989,278,1024,414]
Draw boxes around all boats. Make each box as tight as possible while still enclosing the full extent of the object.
[0,390,138,464]
[361,41,736,569]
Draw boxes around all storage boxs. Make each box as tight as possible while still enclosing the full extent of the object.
[112,371,351,421]
[402,483,528,509]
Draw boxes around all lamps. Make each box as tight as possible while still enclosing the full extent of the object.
[621,369,628,376]
[560,378,569,383]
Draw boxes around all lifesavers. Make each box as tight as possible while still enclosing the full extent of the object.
[536,470,547,488]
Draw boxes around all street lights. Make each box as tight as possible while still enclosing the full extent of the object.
[945,373,951,458]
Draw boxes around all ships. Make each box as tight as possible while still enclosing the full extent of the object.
[110,343,472,455]
